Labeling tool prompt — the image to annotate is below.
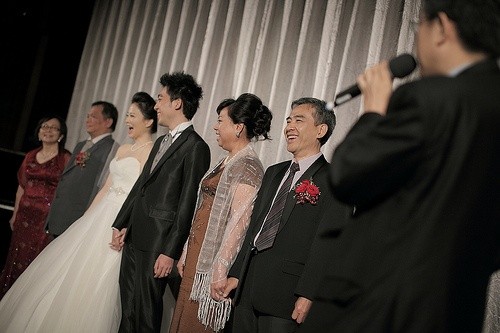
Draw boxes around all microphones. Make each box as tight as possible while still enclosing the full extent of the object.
[334,53,417,108]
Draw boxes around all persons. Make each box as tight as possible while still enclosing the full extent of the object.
[108,70,211,333]
[43,101,120,243]
[325,0,500,333]
[52,92,158,333]
[221,97,337,333]
[0,115,73,302]
[166,92,274,333]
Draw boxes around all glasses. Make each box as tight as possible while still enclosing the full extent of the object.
[41,125,60,131]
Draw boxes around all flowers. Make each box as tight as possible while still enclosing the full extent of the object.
[76,151,94,167]
[292,177,321,206]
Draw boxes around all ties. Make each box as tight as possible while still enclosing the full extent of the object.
[150,133,173,174]
[255,162,300,251]
